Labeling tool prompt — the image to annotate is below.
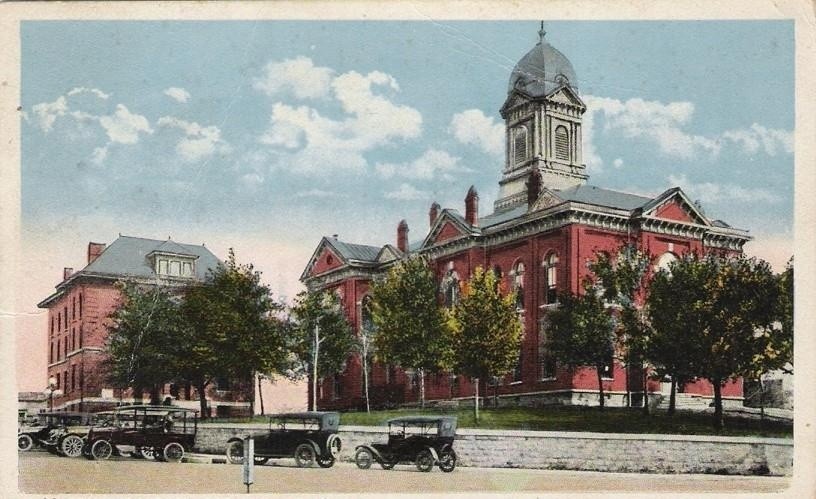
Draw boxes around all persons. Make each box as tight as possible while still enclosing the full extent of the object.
[162,395,176,418]
[18,420,26,432]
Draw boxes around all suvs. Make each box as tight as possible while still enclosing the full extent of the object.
[352,415,461,472]
[224,409,342,470]
[17,400,201,465]
